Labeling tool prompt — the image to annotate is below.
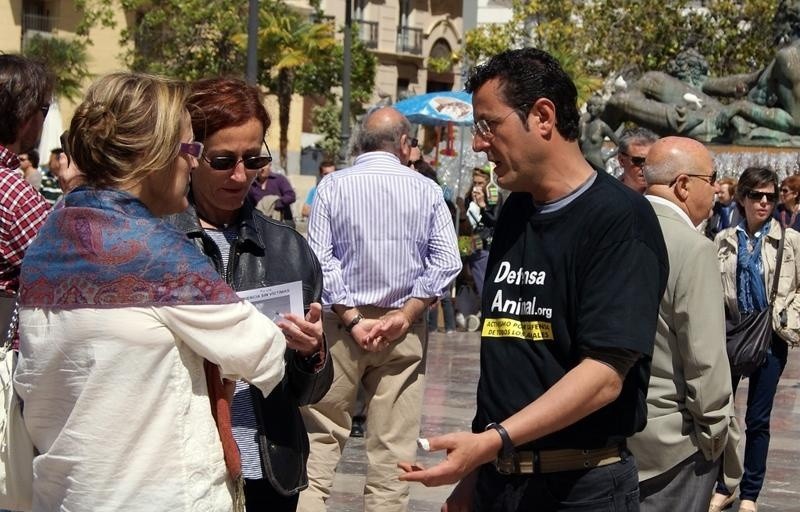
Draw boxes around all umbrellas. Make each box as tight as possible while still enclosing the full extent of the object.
[393,87,474,169]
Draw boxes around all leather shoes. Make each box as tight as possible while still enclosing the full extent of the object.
[351,421,364,436]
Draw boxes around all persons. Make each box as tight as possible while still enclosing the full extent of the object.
[296,105,466,511]
[1,52,58,511]
[576,94,619,170]
[11,145,798,334]
[708,163,799,509]
[15,75,287,511]
[161,76,337,511]
[598,0,800,143]
[626,136,745,511]
[396,48,671,511]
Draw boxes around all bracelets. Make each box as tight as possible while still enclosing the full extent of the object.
[480,419,515,463]
[343,312,368,338]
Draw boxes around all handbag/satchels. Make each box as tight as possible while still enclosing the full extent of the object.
[0,349,35,512]
[726,304,773,372]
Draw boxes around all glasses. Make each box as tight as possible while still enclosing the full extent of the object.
[670,170,717,187]
[182,142,203,161]
[471,104,528,135]
[475,167,489,176]
[747,190,779,202]
[18,157,27,160]
[37,103,49,117]
[406,136,418,147]
[203,139,272,170]
[617,151,646,166]
[780,188,788,193]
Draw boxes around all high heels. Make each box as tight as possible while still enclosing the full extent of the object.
[738,500,757,512]
[708,491,736,512]
